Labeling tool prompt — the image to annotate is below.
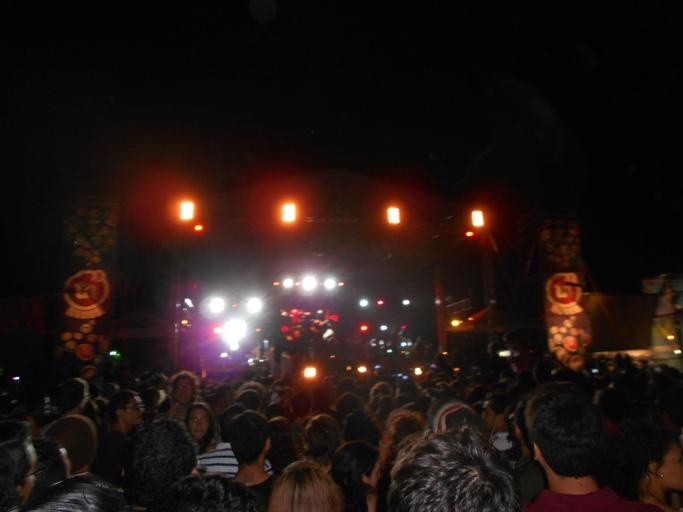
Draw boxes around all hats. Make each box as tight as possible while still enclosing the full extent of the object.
[51,378,90,411]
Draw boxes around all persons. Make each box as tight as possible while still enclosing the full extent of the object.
[386,353,680,502]
[2,370,392,512]
[519,383,663,512]
[615,427,679,512]
[386,429,520,512]
